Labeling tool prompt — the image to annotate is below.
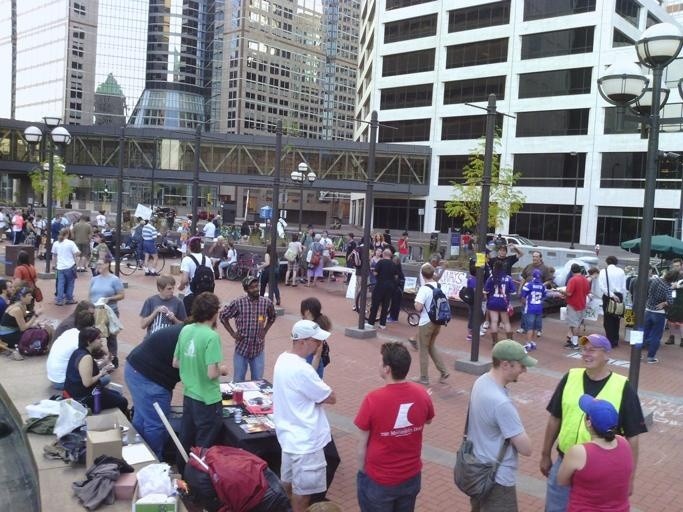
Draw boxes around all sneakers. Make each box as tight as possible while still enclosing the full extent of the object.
[466,320,543,352]
[150,271,159,276]
[55,301,63,306]
[411,376,429,385]
[285,277,336,287]
[647,359,658,363]
[144,272,150,276]
[439,373,449,382]
[364,317,399,329]
[65,299,78,304]
[563,342,578,349]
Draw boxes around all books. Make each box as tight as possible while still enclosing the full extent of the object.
[235,411,275,433]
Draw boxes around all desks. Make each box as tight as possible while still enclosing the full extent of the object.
[216,378,282,471]
[321,265,354,289]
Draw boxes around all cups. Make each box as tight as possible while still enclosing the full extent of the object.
[232,409,243,424]
[231,389,243,405]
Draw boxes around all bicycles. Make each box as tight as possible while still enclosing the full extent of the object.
[225,249,260,282]
[117,237,164,276]
[348,280,426,328]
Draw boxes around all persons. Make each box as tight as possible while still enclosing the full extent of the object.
[176,445,292,512]
[540,334,648,511]
[171,291,229,474]
[272,319,336,511]
[124,319,218,462]
[557,395,634,511]
[354,342,436,512]
[468,339,538,511]
[1,206,683,411]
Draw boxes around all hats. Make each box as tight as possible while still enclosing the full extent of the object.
[289,319,331,340]
[578,333,611,352]
[491,338,537,367]
[578,393,618,434]
[241,275,259,287]
[533,269,542,283]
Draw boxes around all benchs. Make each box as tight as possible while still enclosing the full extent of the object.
[110,230,182,259]
[203,241,287,278]
[509,272,566,317]
[402,262,470,316]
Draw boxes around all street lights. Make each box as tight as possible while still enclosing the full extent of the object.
[290,161,314,240]
[598,21,683,396]
[24,116,69,267]
[564,147,582,249]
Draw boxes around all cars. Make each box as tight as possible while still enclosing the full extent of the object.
[471,231,531,260]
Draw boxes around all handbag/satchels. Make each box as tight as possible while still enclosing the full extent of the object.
[458,286,474,305]
[33,287,43,302]
[283,249,297,263]
[453,441,496,500]
[605,299,625,316]
[506,304,514,317]
[18,322,50,357]
[310,251,321,266]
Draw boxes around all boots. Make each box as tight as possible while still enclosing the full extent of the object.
[680,338,683,346]
[665,335,674,344]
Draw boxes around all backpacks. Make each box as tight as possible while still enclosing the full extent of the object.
[43,424,86,465]
[424,283,451,327]
[186,253,215,293]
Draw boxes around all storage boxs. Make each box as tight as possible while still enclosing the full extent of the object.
[121,443,156,473]
[132,473,182,512]
[86,413,122,466]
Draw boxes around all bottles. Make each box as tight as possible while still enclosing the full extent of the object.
[92,385,102,414]
